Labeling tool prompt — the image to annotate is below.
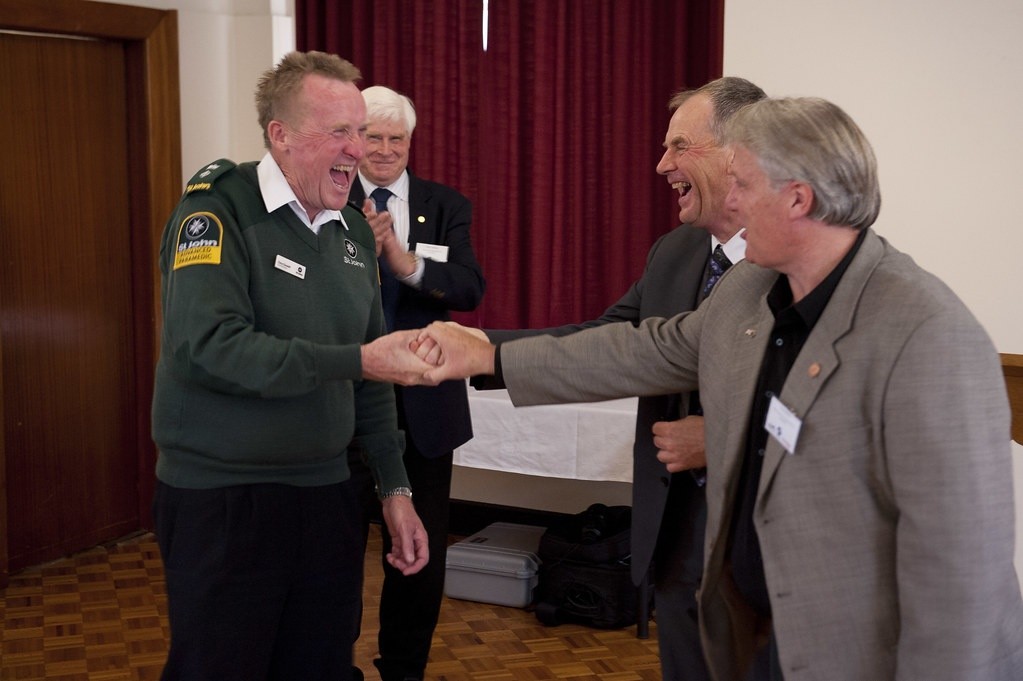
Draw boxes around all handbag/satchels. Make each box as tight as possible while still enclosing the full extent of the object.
[533,502,660,632]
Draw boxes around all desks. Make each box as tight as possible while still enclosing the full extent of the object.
[449,376,654,640]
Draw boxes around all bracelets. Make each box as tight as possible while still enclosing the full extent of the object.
[384,487,412,497]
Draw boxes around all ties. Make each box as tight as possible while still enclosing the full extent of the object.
[703,246,733,300]
[372,188,396,273]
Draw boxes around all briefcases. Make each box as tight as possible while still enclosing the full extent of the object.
[443,521,548,609]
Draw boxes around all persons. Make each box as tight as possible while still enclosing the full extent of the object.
[410,78,768,681]
[150,50,441,681]
[346,87,483,681]
[416,98,1023,680]
